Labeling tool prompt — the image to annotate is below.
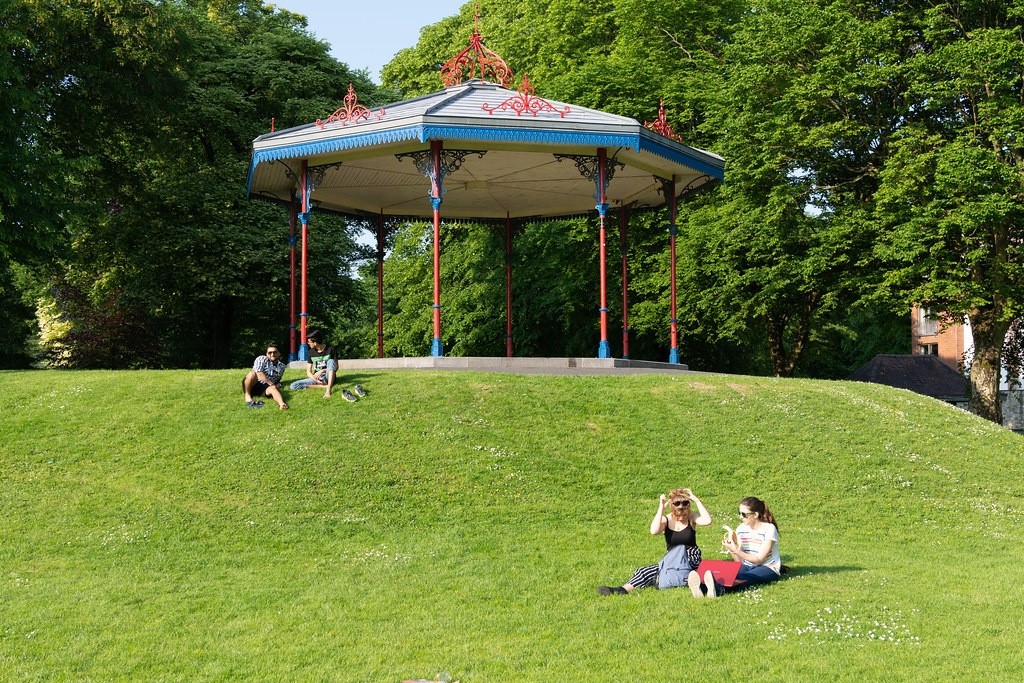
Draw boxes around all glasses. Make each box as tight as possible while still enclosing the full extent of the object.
[738,511,755,518]
[268,351,278,354]
[672,500,689,506]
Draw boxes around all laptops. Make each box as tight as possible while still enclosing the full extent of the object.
[697,560,747,587]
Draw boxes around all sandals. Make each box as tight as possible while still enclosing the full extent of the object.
[248,401,265,408]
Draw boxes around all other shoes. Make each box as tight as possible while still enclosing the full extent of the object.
[704,570,723,599]
[599,586,628,596]
[687,570,704,598]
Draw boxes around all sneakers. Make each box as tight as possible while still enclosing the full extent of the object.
[355,385,368,397]
[342,390,358,402]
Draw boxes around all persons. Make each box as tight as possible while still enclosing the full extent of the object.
[242,341,288,411]
[688,497,781,600]
[597,488,711,595]
[290,330,339,397]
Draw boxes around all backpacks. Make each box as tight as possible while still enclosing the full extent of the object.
[654,545,692,589]
